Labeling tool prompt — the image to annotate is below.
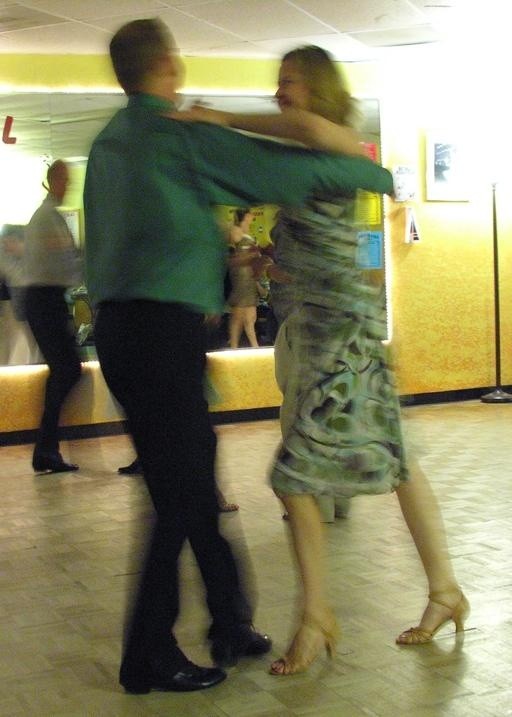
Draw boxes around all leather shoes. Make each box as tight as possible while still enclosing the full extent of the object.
[119,458,141,473]
[216,500,239,512]
[32,453,78,472]
[124,661,226,695]
[211,634,270,668]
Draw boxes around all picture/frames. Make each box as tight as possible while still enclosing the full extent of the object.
[56,208,81,249]
[425,124,470,202]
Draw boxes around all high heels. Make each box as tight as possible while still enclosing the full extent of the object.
[268,612,338,677]
[396,587,470,645]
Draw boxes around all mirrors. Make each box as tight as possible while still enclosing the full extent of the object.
[0,85,392,374]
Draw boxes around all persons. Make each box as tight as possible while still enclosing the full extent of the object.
[114,455,141,476]
[21,160,84,473]
[78,13,397,696]
[0,223,40,367]
[217,484,241,512]
[268,223,352,523]
[227,208,263,349]
[157,41,472,676]
